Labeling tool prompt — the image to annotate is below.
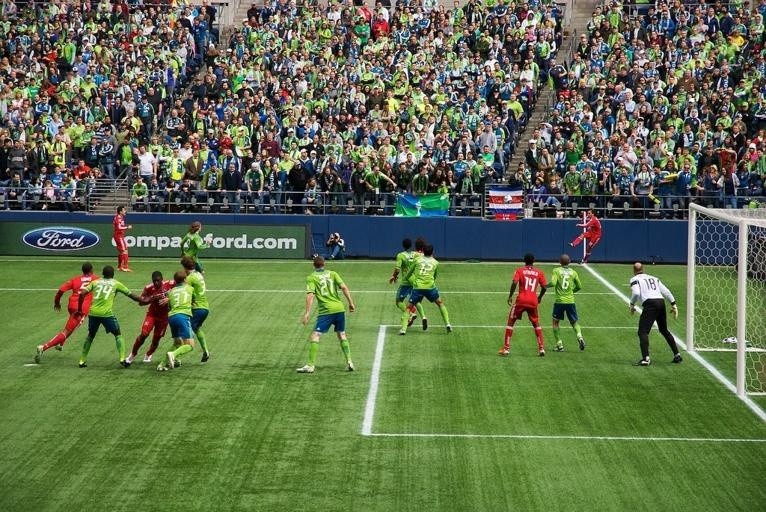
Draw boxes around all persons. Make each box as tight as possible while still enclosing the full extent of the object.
[389,237,452,334]
[568,209,602,264]
[629,263,683,366]
[326,233,346,260]
[2,1,766,219]
[546,254,585,352]
[498,254,548,355]
[35,257,210,371]
[113,206,133,272]
[180,222,211,276]
[296,256,355,373]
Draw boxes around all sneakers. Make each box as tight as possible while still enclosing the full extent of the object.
[579,337,585,350]
[56,344,62,350]
[157,351,181,371]
[297,365,314,373]
[553,346,564,352]
[400,328,406,334]
[672,355,682,363]
[78,361,87,368]
[117,267,131,272]
[537,350,544,356]
[408,314,417,326]
[201,352,210,362]
[120,353,135,367]
[35,345,43,363]
[348,363,354,370]
[639,359,651,365]
[498,348,510,354]
[143,353,152,363]
[422,317,427,330]
[446,324,452,332]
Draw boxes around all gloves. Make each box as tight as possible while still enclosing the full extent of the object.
[630,305,636,314]
[670,305,678,319]
